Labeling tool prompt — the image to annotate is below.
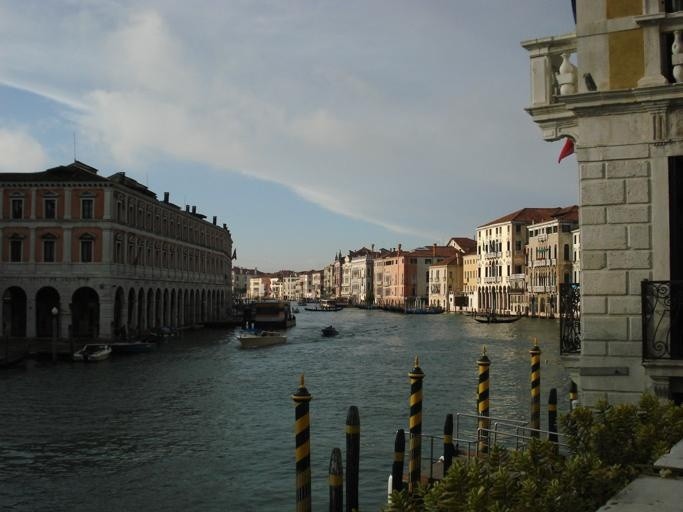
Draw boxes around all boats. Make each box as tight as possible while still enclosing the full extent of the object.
[237,330,288,347]
[473,315,523,325]
[71,340,113,362]
[301,304,343,313]
[322,322,337,339]
[353,302,444,315]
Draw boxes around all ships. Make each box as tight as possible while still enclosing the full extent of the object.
[245,300,300,328]
